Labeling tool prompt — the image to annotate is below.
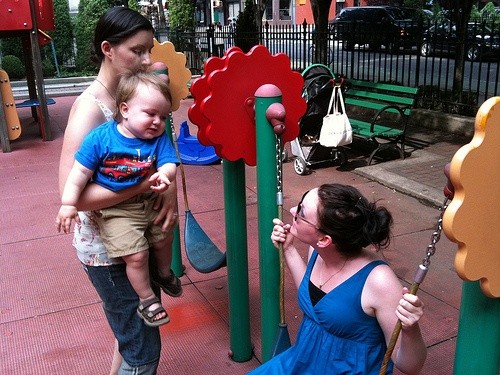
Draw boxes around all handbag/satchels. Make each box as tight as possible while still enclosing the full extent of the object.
[319,86,352,148]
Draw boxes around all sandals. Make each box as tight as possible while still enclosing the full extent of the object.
[136,297,170,327]
[152,269,183,297]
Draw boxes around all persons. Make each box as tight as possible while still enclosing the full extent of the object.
[59,6,180,375]
[54,69,183,327]
[214,21,225,57]
[244,183,427,375]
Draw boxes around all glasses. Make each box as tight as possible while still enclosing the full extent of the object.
[295,190,337,244]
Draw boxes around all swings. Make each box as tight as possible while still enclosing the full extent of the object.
[273,131,448,375]
[169,112,227,274]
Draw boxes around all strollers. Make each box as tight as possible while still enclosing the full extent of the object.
[279,63,352,174]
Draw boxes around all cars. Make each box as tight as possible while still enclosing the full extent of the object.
[329,5,432,53]
[424,21,497,59]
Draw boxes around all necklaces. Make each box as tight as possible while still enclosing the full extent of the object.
[95,78,116,102]
[319,258,347,290]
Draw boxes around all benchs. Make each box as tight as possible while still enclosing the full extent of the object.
[345,78,419,166]
